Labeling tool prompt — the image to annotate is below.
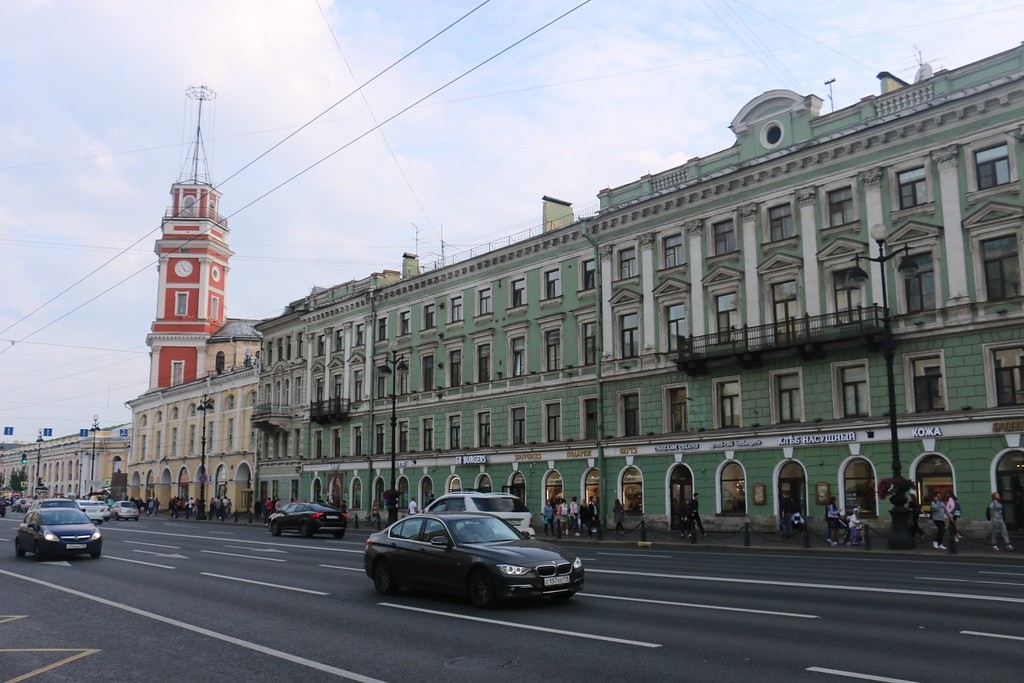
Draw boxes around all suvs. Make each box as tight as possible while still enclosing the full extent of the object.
[411,487,535,542]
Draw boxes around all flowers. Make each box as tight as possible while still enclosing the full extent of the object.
[878,477,916,500]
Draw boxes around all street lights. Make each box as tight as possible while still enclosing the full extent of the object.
[196,392,215,521]
[35,431,44,498]
[377,337,408,527]
[847,223,921,550]
[89,414,101,493]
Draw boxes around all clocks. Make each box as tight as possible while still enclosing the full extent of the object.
[175,261,193,277]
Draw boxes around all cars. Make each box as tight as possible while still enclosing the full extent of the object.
[363,512,585,610]
[14,508,103,563]
[266,501,346,540]
[11,499,36,513]
[6,497,18,505]
[0,497,7,517]
[110,501,140,521]
[74,500,111,525]
[28,499,81,513]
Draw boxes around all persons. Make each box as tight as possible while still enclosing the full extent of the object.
[130,496,280,524]
[341,492,1016,552]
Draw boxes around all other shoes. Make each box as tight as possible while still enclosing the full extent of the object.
[932,541,939,548]
[939,545,947,550]
[992,546,1000,552]
[833,542,838,545]
[574,533,580,536]
[1007,545,1015,551]
[827,538,832,543]
[566,529,569,536]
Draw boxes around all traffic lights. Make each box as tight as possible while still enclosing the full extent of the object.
[38,478,43,486]
[22,454,28,464]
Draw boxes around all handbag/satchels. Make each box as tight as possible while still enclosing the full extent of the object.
[954,510,960,517]
[568,503,573,513]
